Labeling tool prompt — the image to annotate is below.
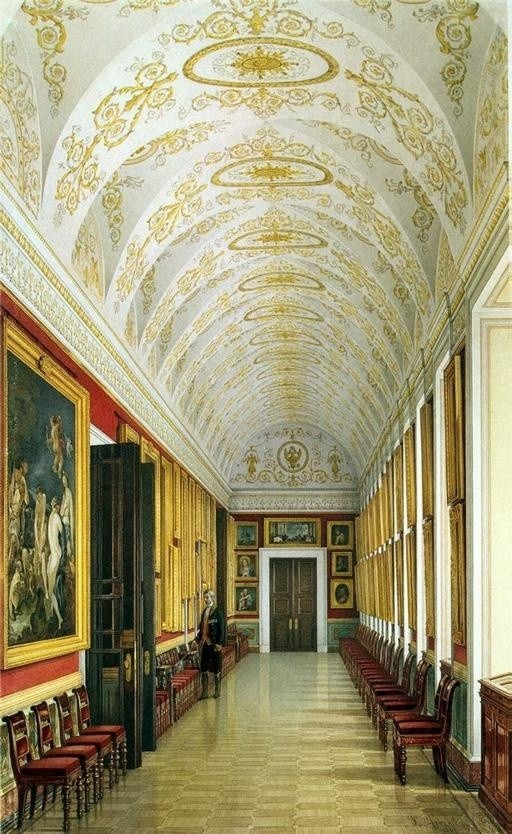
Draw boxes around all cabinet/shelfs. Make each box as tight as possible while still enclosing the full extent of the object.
[477,672,512,834]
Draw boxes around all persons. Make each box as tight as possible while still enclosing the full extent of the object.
[241,559,251,577]
[190,590,227,700]
[8,415,75,630]
[335,528,345,545]
[239,589,253,611]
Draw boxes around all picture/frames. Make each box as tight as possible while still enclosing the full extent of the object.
[0,316,91,671]
[120,424,217,638]
[327,521,354,550]
[354,355,467,647]
[234,551,258,581]
[233,521,258,549]
[332,552,352,576]
[330,579,354,609]
[233,583,259,615]
[264,518,321,548]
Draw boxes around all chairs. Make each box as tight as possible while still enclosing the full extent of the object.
[338,623,460,784]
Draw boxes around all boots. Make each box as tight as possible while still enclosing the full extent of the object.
[198,673,209,700]
[212,674,222,698]
